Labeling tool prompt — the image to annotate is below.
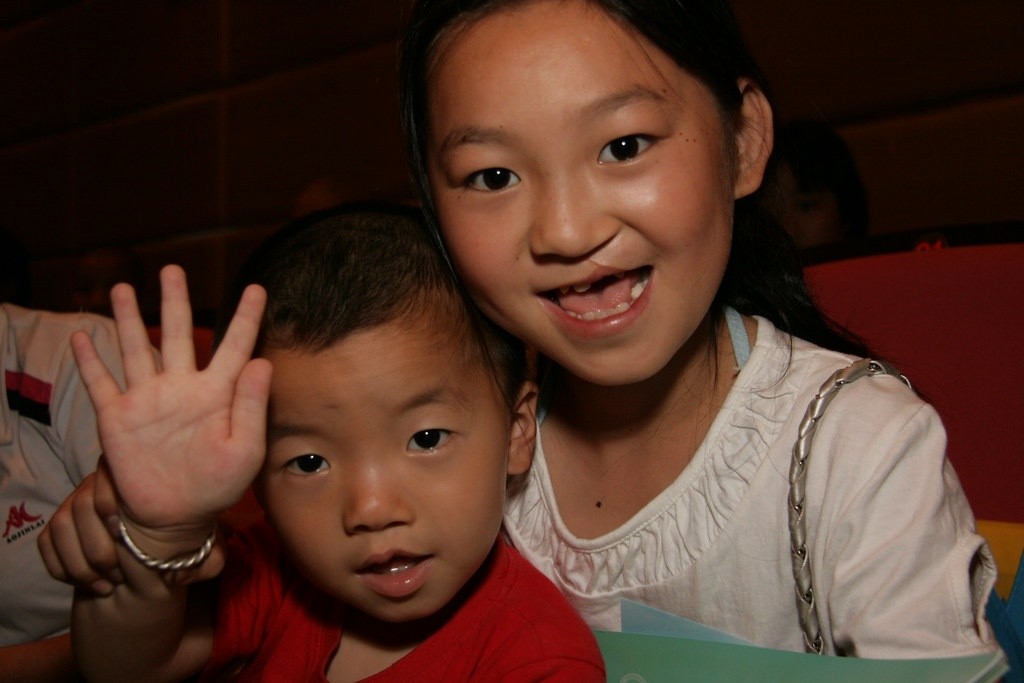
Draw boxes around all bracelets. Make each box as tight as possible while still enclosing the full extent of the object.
[120,517,218,570]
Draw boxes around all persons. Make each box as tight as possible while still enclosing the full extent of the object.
[37,0,994,682]
[70,203,605,683]
[0,304,162,682]
